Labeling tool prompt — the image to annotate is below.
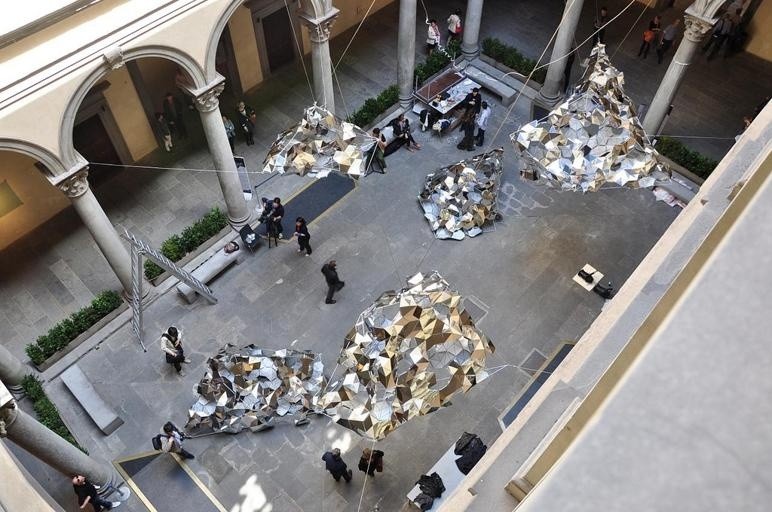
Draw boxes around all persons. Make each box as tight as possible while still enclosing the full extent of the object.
[153,90,257,152]
[392,113,422,153]
[633,7,744,65]
[70,474,121,512]
[257,197,285,241]
[292,216,314,257]
[151,433,195,460]
[320,258,346,304]
[456,83,491,151]
[159,325,192,376]
[446,9,463,46]
[425,18,442,56]
[320,447,353,483]
[592,8,608,47]
[164,421,184,448]
[370,127,388,173]
[357,447,386,477]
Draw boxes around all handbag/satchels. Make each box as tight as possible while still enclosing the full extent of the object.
[376,455,383,473]
[166,349,185,363]
[248,115,257,127]
[455,25,463,32]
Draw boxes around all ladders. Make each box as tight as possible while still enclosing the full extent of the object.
[115,222,218,352]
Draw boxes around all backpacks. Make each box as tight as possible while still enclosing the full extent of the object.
[152,434,169,451]
[223,240,239,254]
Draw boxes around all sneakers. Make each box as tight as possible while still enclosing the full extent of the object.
[178,369,186,377]
[184,358,192,364]
[186,453,194,460]
[468,147,476,151]
[407,147,414,152]
[111,502,120,508]
[348,469,352,480]
[383,168,387,172]
[457,144,465,150]
[414,142,420,148]
[476,142,482,146]
[325,300,336,304]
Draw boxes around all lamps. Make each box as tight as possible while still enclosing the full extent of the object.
[180,342,329,439]
[509,40,673,193]
[259,103,387,183]
[417,146,506,242]
[313,268,496,444]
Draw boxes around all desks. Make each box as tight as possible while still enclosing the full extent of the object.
[404,434,488,512]
